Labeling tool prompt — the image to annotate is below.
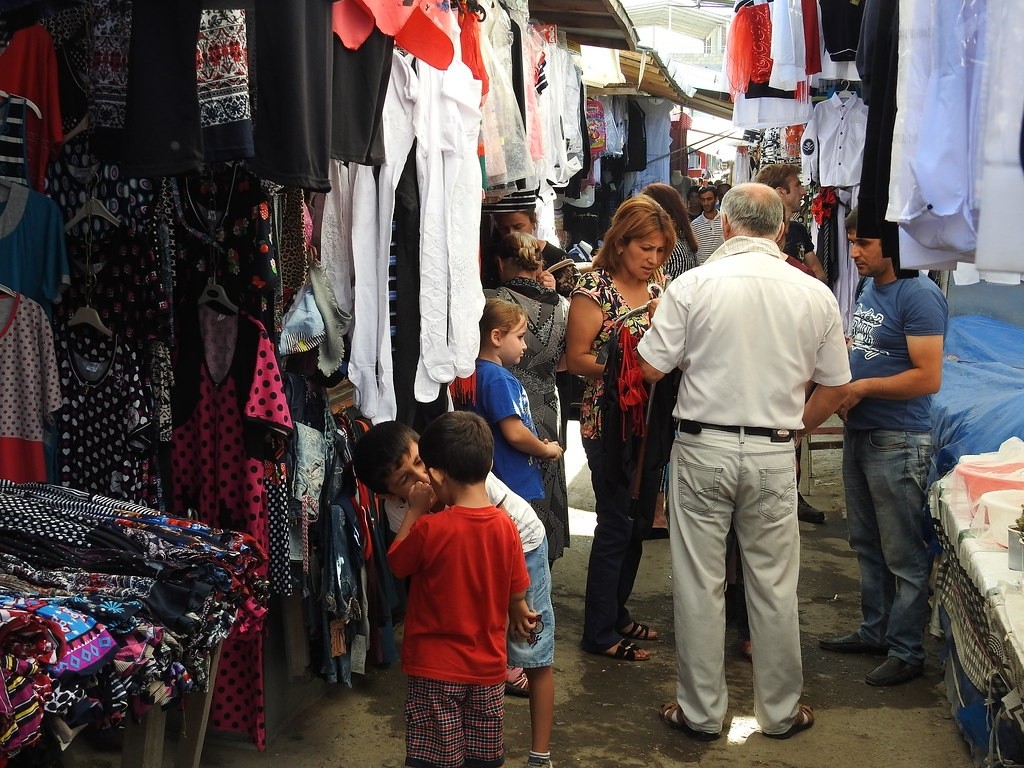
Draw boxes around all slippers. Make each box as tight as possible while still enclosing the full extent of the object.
[618,621,659,640]
[602,639,651,660]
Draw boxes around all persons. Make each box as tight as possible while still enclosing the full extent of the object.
[353,421,556,768]
[640,183,699,282]
[466,298,563,699]
[565,192,683,661]
[385,410,530,768]
[482,231,571,571]
[819,205,949,687]
[754,164,827,285]
[685,180,732,266]
[636,181,852,743]
[492,204,582,453]
[568,240,593,263]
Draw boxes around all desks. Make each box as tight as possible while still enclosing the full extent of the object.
[929,475,1024,768]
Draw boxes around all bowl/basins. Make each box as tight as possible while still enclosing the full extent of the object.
[956,463,1024,524]
[980,488,1024,549]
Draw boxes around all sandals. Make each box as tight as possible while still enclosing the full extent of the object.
[762,704,814,739]
[660,702,721,741]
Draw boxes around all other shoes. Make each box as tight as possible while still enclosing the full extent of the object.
[742,641,752,661]
[504,668,529,696]
[526,758,552,768]
[798,492,825,523]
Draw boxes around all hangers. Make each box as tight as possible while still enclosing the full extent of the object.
[61,109,89,146]
[198,261,238,314]
[837,79,854,100]
[64,170,120,235]
[65,270,114,339]
[0,89,42,120]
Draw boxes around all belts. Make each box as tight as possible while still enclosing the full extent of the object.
[675,419,771,436]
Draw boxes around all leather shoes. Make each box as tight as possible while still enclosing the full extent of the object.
[864,657,923,686]
[819,631,890,656]
